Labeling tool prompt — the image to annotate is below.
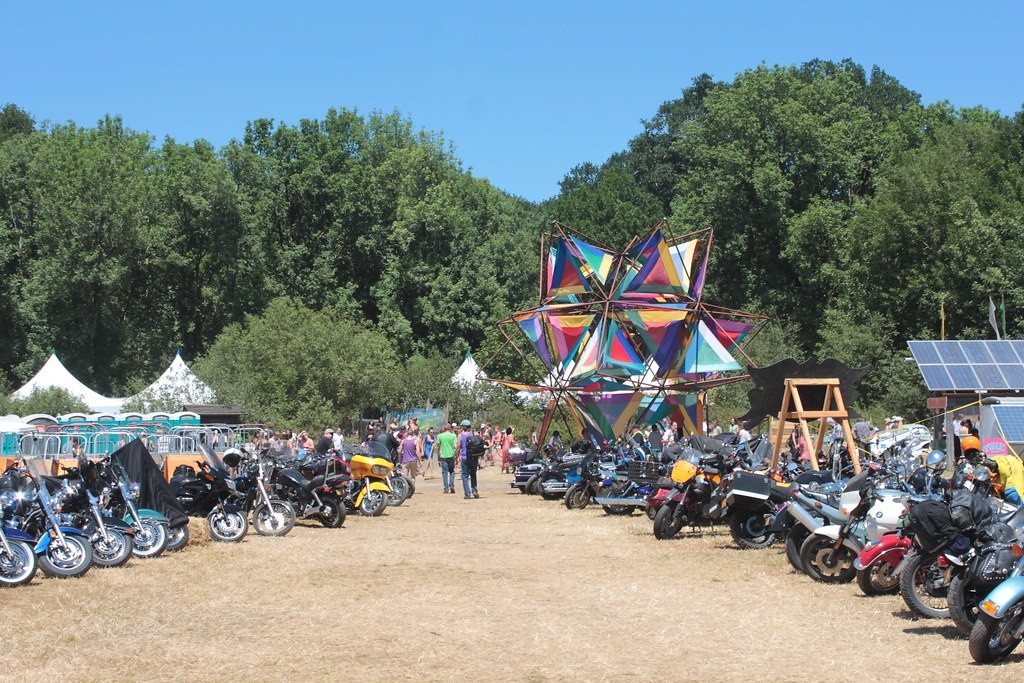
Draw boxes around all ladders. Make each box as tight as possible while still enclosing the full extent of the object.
[771,378,861,476]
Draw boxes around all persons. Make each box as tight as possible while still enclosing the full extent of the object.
[529,425,538,446]
[436,420,480,499]
[906,447,987,493]
[827,422,843,443]
[69,435,84,455]
[702,417,753,444]
[477,422,520,474]
[420,427,437,478]
[115,422,345,461]
[788,423,810,464]
[828,436,849,468]
[366,415,424,484]
[953,415,981,441]
[581,427,590,440]
[851,418,876,461]
[549,431,562,454]
[631,421,677,460]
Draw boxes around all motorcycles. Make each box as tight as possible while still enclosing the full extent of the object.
[506,433,1024,665]
[1,440,416,588]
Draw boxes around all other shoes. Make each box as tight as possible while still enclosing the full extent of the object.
[445,490,449,494]
[473,492,479,498]
[450,486,455,494]
[464,495,470,499]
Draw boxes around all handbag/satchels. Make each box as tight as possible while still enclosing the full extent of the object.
[787,430,797,447]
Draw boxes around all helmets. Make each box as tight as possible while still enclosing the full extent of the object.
[223,448,244,468]
[962,436,981,452]
[927,450,945,465]
[461,420,471,427]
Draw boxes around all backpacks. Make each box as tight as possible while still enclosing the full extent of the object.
[908,498,962,555]
[467,435,485,458]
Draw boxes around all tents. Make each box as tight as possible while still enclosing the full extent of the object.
[6,349,219,414]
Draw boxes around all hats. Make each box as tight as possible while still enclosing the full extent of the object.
[794,423,800,428]
[324,429,336,433]
[632,424,640,429]
[481,423,486,428]
[892,415,897,418]
[884,418,891,422]
[895,416,903,421]
